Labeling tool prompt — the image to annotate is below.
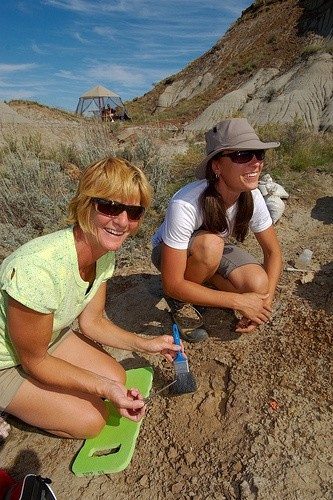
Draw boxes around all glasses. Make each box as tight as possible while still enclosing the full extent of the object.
[93,198,145,221]
[220,150,265,162]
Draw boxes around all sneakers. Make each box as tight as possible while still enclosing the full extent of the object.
[171,303,209,342]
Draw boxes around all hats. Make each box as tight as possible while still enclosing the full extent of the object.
[195,117,281,179]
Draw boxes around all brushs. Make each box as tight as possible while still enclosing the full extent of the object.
[172,325,198,395]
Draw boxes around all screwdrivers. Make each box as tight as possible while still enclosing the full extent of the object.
[129,380,178,416]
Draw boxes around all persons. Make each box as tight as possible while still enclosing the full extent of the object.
[0,154,188,440]
[152,118,283,342]
[102,104,116,122]
